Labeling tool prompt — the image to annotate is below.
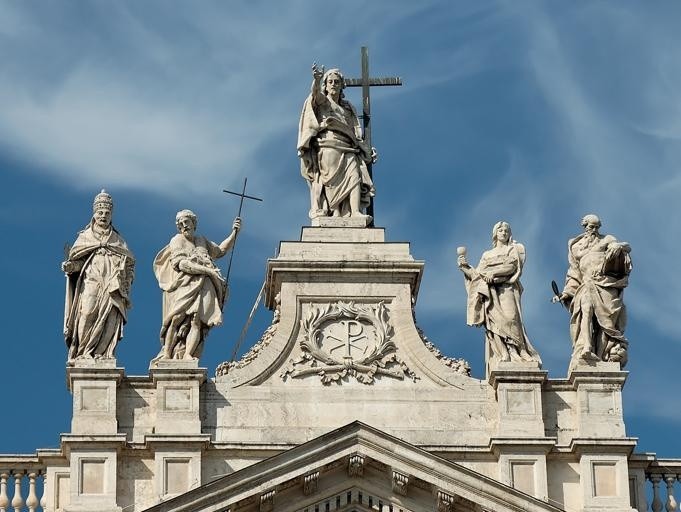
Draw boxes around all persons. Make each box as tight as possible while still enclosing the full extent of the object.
[152,208,243,360]
[295,61,377,221]
[549,213,635,367]
[457,221,545,366]
[60,189,138,367]
